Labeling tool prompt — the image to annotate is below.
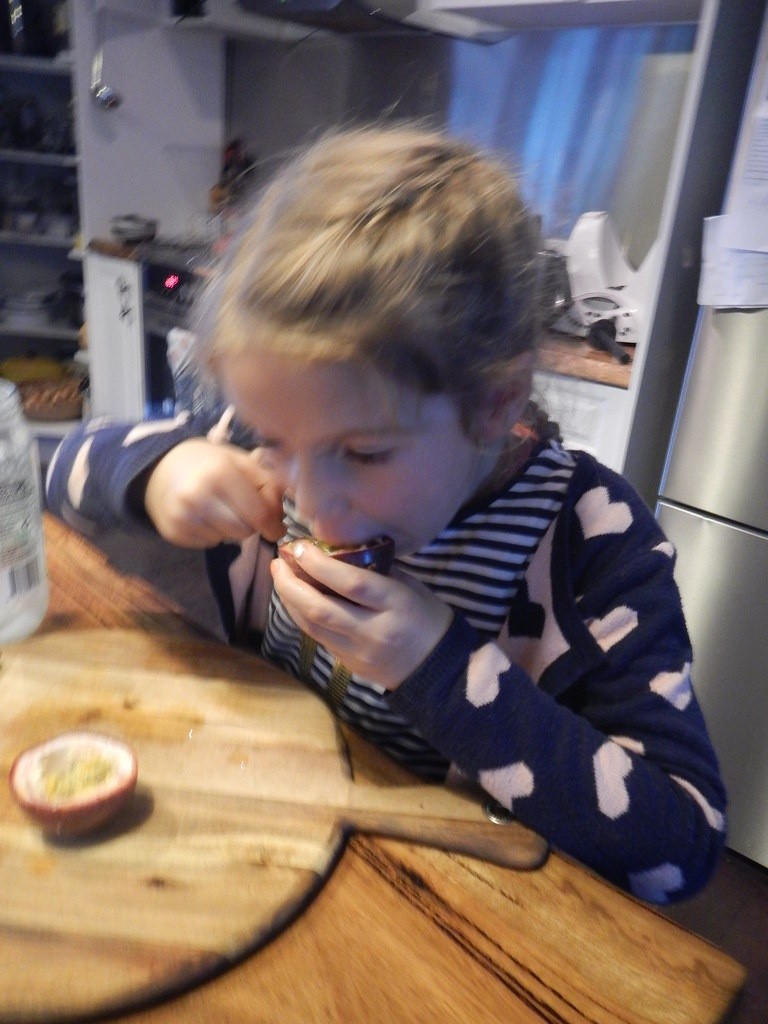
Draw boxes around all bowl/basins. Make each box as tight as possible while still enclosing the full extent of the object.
[9,731,138,839]
[277,535,394,605]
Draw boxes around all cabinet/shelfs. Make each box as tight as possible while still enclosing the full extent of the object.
[0,52,89,475]
[79,238,149,425]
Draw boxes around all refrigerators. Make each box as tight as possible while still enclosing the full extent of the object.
[655,10,767,869]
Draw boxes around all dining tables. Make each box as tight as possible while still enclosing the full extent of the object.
[0,503,750,1024]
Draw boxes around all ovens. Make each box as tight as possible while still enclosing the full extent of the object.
[140,263,205,416]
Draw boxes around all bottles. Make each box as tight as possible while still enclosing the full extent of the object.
[0,379,49,647]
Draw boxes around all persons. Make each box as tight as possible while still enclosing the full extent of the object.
[46,126,727,905]
